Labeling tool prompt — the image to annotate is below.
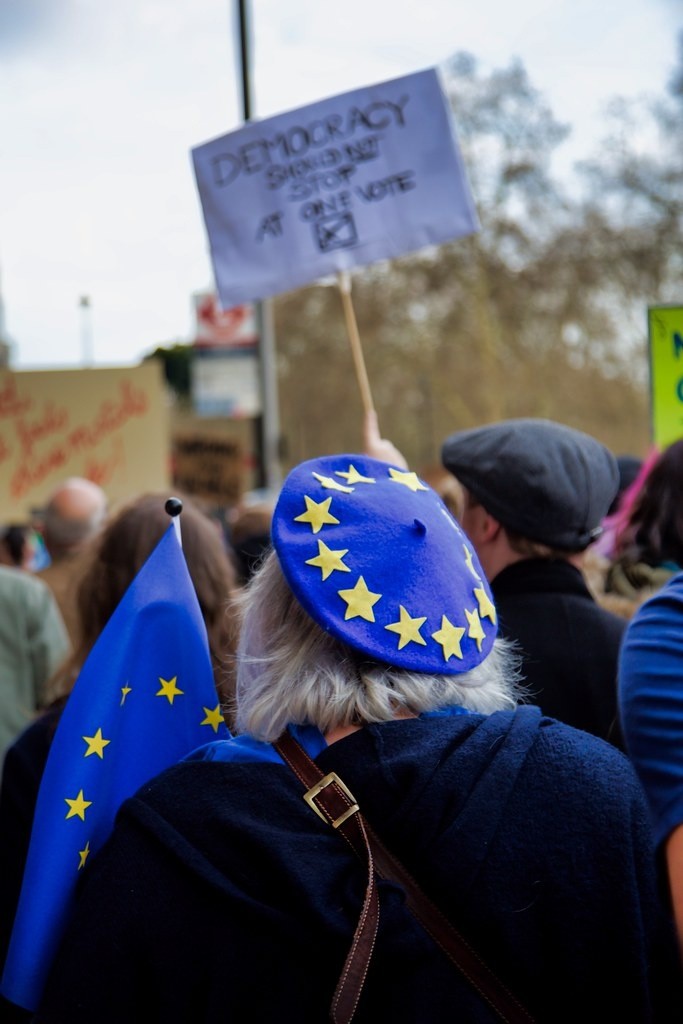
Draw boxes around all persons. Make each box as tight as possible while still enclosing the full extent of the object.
[49,454,683,1024]
[365,420,683,940]
[0,478,274,970]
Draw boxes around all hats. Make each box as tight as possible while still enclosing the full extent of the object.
[269,454,497,675]
[440,419,619,553]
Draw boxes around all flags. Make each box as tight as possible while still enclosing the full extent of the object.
[4,524,228,1011]
